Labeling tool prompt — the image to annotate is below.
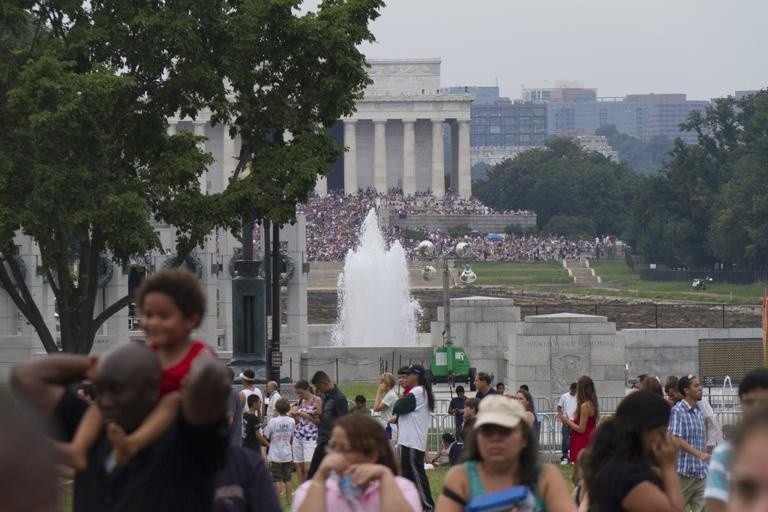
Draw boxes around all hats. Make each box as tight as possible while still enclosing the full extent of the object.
[472,394,529,432]
[402,363,425,374]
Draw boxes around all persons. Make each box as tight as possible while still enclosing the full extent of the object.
[666,374,709,511]
[51,268,213,470]
[218,369,239,432]
[516,388,540,451]
[350,396,371,414]
[396,365,413,396]
[289,380,322,480]
[263,381,281,410]
[292,412,424,512]
[393,364,439,511]
[238,370,262,430]
[706,363,767,511]
[375,373,402,441]
[639,377,664,412]
[434,432,456,468]
[450,386,473,433]
[263,399,297,508]
[310,370,349,479]
[368,189,528,216]
[729,408,768,511]
[461,398,484,459]
[303,188,380,260]
[472,374,497,400]
[663,375,721,456]
[495,381,506,394]
[13,339,229,511]
[380,223,615,260]
[558,383,577,465]
[582,388,696,512]
[436,396,575,512]
[560,376,598,484]
[239,395,265,450]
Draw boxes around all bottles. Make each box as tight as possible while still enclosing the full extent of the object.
[340,468,370,511]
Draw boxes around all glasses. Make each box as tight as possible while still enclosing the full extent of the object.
[686,372,696,385]
